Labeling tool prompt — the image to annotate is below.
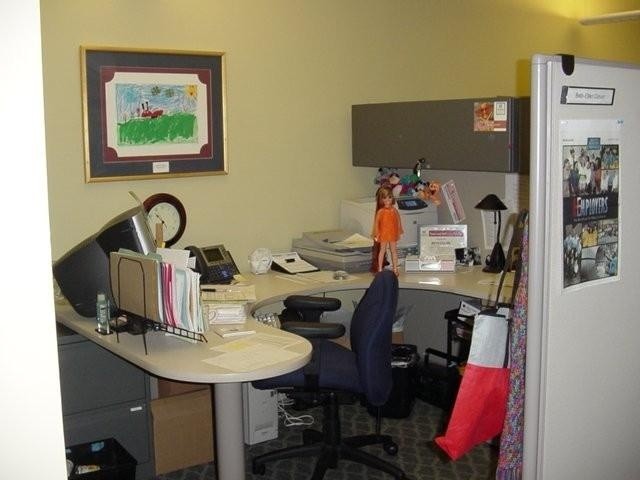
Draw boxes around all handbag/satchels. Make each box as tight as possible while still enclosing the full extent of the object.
[435,300,511,461]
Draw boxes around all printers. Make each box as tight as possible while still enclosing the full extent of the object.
[341,194,438,247]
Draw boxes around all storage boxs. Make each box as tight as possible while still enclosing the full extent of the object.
[146,377,214,477]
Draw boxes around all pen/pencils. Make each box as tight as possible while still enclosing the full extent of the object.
[201,289,240,293]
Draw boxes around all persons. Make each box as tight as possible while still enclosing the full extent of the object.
[373,186,404,279]
[563,144,620,287]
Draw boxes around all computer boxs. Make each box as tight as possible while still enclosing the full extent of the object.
[242,382,279,446]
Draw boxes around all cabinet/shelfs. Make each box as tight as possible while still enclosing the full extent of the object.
[56,332,157,480]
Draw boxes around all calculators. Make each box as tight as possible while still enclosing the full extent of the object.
[272,252,319,274]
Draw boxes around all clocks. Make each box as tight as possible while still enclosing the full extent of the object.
[141,193,187,249]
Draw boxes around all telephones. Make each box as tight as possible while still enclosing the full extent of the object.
[185,244,240,285]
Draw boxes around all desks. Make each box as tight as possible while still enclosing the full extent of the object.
[54,262,524,479]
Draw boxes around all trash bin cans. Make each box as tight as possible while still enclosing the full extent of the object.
[366,344,417,419]
[65,437,137,480]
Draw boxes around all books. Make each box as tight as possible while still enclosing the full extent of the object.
[109,248,206,332]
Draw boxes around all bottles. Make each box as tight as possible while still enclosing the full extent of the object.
[96,294,108,333]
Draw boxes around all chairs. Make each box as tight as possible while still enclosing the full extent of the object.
[252,271,403,480]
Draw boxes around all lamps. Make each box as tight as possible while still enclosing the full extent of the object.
[473,193,508,274]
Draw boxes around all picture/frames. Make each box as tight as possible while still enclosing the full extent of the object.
[79,45,229,184]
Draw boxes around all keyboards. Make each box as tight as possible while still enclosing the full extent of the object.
[254,312,281,329]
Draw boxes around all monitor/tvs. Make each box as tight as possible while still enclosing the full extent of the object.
[52,205,157,317]
